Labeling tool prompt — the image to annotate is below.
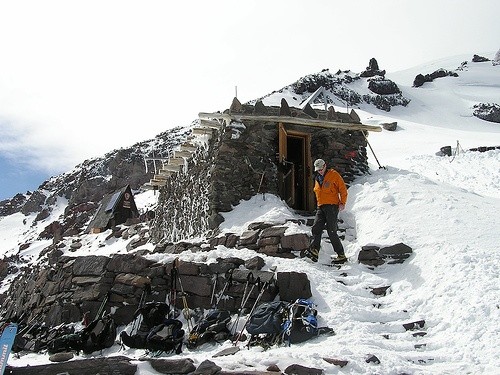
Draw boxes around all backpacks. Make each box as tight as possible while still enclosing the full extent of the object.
[119,301,184,354]
[188,308,231,347]
[283,299,318,344]
[0,315,117,355]
[246,301,287,334]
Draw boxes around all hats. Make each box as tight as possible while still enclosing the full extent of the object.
[314,159,325,171]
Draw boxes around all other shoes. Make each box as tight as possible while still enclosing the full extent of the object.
[304,248,319,262]
[331,251,347,264]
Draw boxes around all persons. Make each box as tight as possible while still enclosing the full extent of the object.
[303,159,348,264]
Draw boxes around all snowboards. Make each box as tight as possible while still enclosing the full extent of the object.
[0,322,17,375]
